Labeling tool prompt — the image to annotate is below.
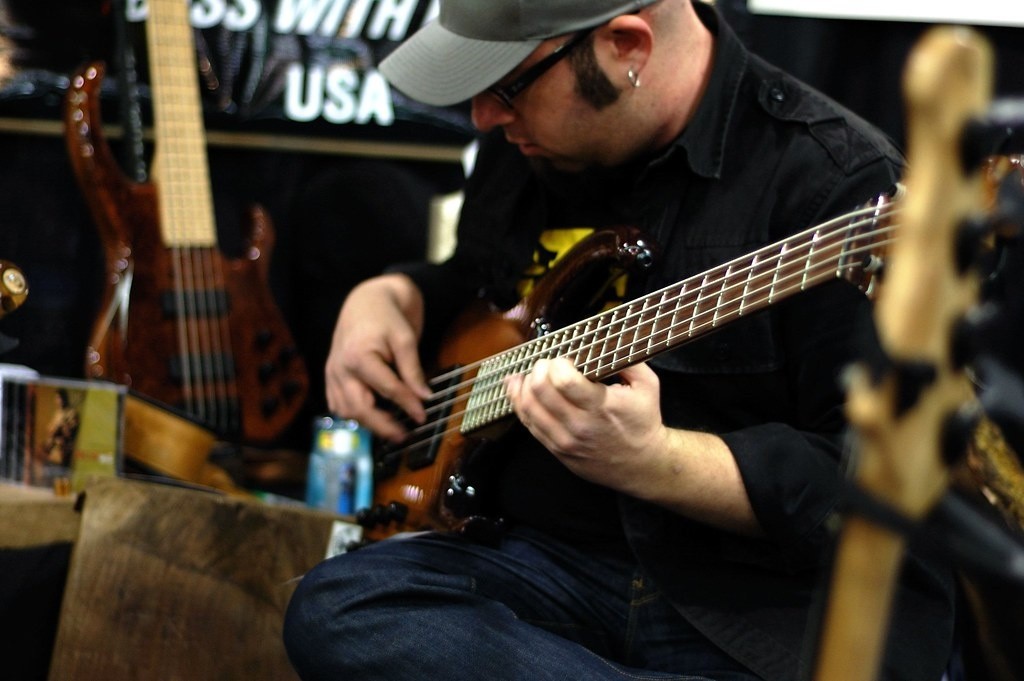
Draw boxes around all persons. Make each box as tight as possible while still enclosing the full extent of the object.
[282,0,906,681]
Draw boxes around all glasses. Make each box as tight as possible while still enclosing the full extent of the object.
[485,28,593,109]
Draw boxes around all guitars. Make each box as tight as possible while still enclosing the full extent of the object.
[66,2,314,444]
[353,152,1017,547]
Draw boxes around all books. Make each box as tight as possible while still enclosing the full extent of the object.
[0,364,127,492]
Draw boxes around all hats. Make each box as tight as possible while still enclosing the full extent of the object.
[376,0,666,108]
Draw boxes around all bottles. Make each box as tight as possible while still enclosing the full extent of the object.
[306,418,374,515]
